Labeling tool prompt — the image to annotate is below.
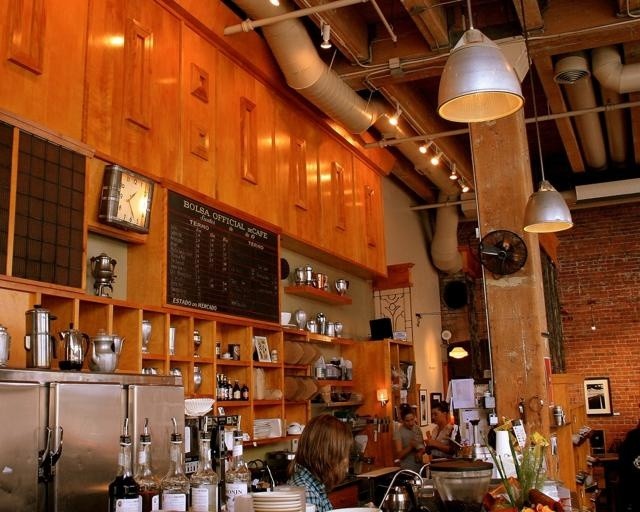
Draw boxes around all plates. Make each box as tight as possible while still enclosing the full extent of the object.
[235,491,306,511]
[252,420,272,438]
[281,324,296,329]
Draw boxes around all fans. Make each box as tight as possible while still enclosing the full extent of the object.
[477,229,528,279]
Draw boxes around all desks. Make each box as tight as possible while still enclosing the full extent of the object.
[593,451,621,512]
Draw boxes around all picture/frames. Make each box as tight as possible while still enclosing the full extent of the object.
[431,392,443,424]
[590,429,607,458]
[420,388,429,427]
[583,378,614,416]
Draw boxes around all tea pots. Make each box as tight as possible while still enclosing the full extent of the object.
[0,305,130,374]
[335,279,349,296]
[295,264,328,291]
[296,309,343,338]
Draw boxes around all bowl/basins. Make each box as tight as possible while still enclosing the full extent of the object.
[331,392,352,402]
[185,398,214,416]
[281,312,293,323]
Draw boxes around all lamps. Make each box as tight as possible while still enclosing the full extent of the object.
[387,102,472,195]
[319,24,333,51]
[437,1,526,125]
[523,43,574,234]
[376,386,389,406]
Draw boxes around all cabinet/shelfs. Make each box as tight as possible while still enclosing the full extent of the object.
[548,373,599,512]
[341,341,422,467]
[329,481,360,510]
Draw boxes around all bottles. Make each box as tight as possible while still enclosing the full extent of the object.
[270,348,279,363]
[552,405,565,425]
[215,371,250,402]
[109,432,248,511]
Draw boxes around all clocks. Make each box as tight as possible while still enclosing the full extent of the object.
[97,165,155,232]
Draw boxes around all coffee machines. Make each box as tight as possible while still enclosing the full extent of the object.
[428,460,493,512]
[265,449,296,486]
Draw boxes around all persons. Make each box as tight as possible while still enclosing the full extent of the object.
[390,402,426,476]
[279,413,355,512]
[424,401,462,460]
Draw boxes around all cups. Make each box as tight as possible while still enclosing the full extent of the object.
[216,341,241,360]
[315,356,353,381]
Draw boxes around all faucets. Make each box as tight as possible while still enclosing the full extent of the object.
[378,469,424,509]
[418,462,430,476]
